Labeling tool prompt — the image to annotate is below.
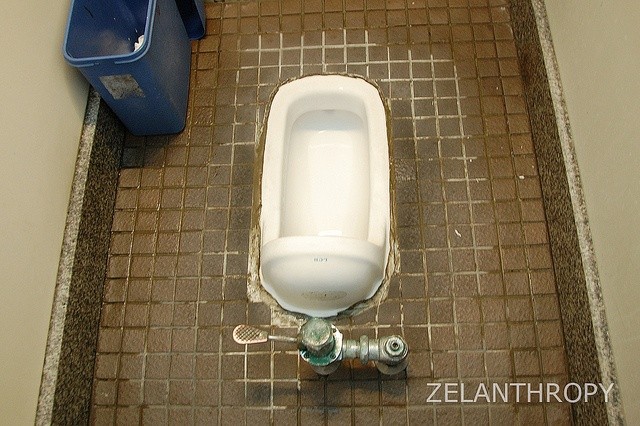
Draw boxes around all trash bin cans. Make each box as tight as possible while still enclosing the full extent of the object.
[63,0,191,137]
